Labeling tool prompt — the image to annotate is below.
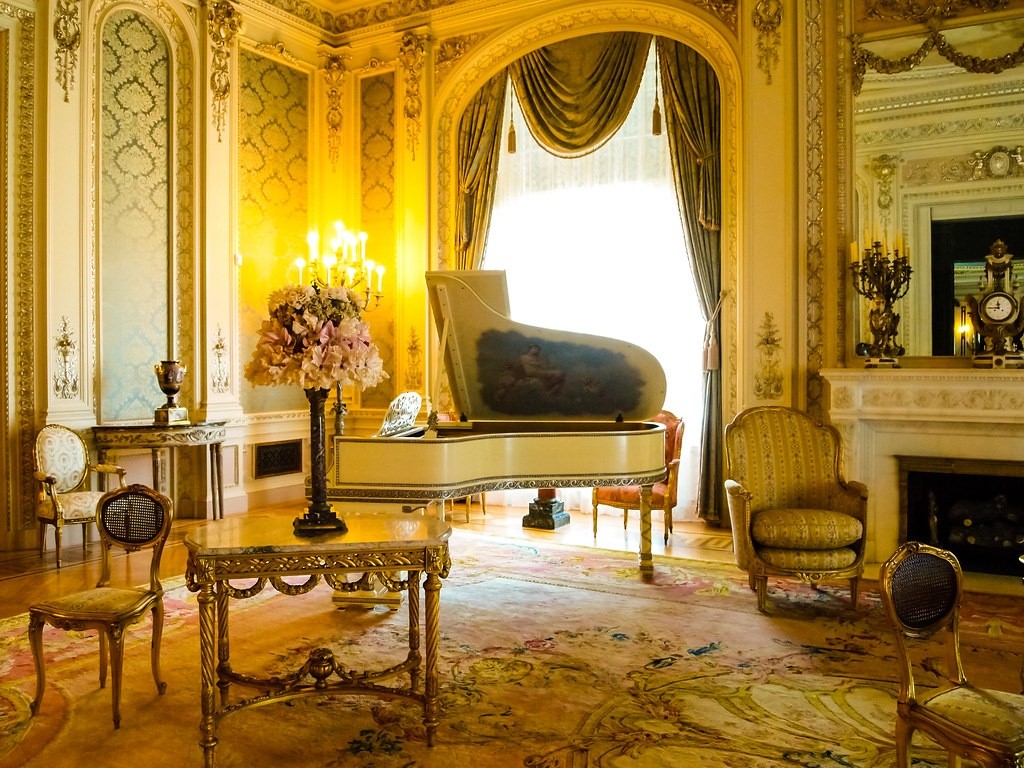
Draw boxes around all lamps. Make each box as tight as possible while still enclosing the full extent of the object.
[152,351,190,428]
[848,241,913,358]
[294,220,385,292]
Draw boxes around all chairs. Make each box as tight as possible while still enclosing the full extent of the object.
[724,406,868,612]
[29,483,174,732]
[592,410,685,546]
[30,424,128,567]
[879,541,1024,768]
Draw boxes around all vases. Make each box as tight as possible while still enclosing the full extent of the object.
[293,389,348,534]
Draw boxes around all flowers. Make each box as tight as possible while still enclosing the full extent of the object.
[244,285,390,393]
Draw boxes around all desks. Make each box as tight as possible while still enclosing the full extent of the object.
[90,421,226,522]
[183,508,452,768]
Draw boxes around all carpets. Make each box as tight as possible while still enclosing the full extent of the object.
[0,527,1024,768]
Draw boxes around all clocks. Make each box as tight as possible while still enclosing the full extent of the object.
[966,239,1024,367]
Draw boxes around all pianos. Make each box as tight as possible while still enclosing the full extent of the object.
[330,267,667,613]
